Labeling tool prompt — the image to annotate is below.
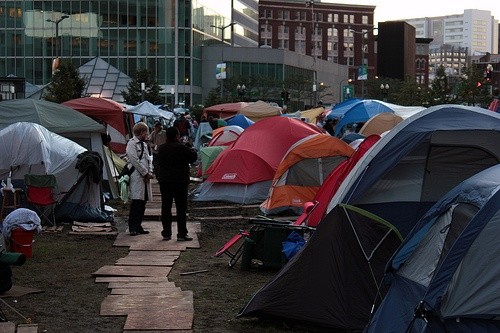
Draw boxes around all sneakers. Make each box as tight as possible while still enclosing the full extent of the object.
[160,229,170,239]
[177,233,193,241]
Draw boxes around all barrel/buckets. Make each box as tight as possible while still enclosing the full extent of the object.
[7,225,33,260]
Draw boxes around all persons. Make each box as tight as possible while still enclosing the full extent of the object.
[151,111,197,149]
[126,121,154,236]
[153,126,198,241]
[317,118,325,127]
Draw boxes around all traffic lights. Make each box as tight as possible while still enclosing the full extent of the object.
[285,90,291,102]
[281,88,285,98]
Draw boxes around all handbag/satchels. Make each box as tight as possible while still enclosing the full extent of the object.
[118,167,130,184]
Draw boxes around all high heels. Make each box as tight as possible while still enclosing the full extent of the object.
[130,230,136,235]
[136,227,149,234]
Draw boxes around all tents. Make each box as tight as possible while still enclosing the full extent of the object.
[0,96,173,201]
[192,99,500,333]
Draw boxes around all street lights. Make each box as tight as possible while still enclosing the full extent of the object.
[350,28,376,100]
[210,22,237,104]
[45,15,70,57]
[237,83,246,102]
[315,82,324,107]
[380,83,390,101]
[340,78,353,104]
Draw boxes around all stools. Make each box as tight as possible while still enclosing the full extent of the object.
[0,188,21,221]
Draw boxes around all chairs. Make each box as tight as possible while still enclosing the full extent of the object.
[216,199,320,270]
[19,172,67,225]
[0,247,32,325]
